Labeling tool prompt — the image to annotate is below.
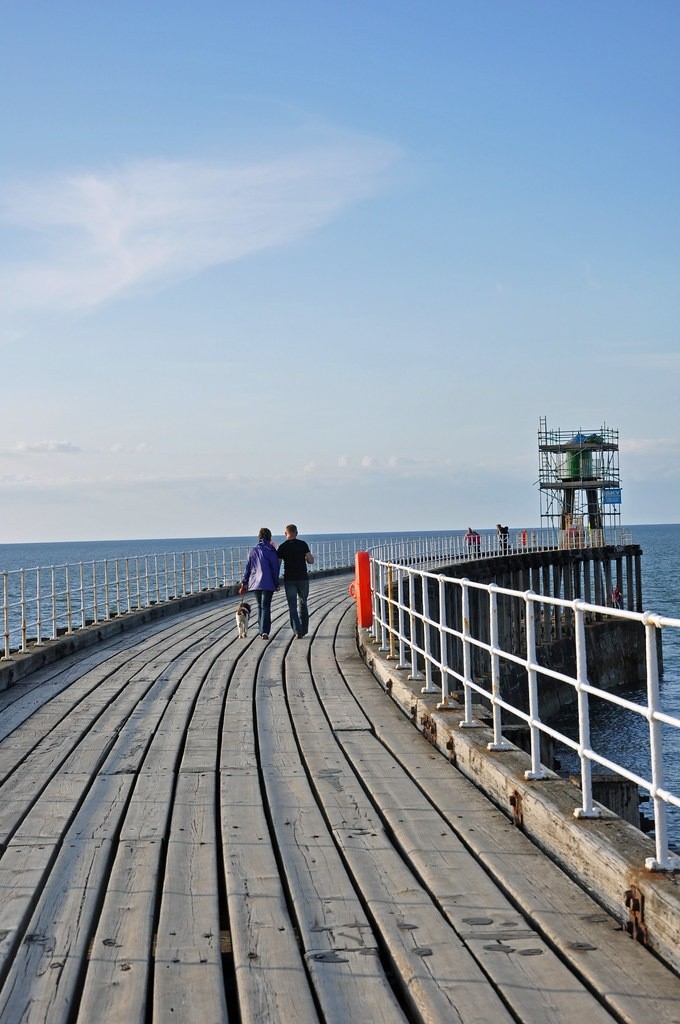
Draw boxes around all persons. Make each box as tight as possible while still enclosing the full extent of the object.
[612,588,622,609]
[464,528,481,559]
[496,524,509,555]
[277,524,314,639]
[239,528,280,639]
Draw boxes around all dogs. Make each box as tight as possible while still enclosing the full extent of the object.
[236,602,251,638]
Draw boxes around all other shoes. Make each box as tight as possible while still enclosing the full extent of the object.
[296,633,303,639]
[260,633,269,640]
